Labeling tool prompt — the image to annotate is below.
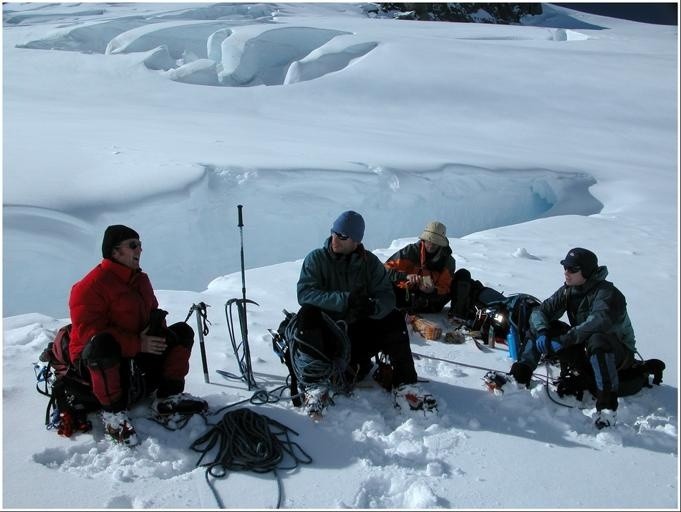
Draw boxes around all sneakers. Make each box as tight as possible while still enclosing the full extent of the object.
[102,409,137,440]
[303,376,329,417]
[405,313,423,328]
[157,394,209,413]
[390,382,439,414]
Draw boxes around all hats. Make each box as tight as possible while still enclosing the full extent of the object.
[331,210,365,241]
[101,224,139,257]
[560,246,599,278]
[419,221,448,247]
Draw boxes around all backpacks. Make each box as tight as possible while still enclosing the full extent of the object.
[453,267,546,351]
[51,323,154,416]
[577,357,665,396]
[274,313,372,396]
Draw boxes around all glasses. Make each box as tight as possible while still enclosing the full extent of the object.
[333,232,349,241]
[130,240,143,248]
[563,266,581,273]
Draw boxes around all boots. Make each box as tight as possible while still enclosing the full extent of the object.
[594,397,619,429]
[482,362,532,392]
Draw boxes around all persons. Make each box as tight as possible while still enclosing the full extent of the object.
[383,217,456,315]
[512,247,637,431]
[450,269,587,363]
[67,224,208,449]
[288,210,440,420]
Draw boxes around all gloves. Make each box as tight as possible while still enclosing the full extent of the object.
[352,297,375,320]
[550,337,561,355]
[347,284,372,309]
[535,331,549,355]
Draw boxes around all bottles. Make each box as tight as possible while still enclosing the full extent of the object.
[506,325,520,362]
